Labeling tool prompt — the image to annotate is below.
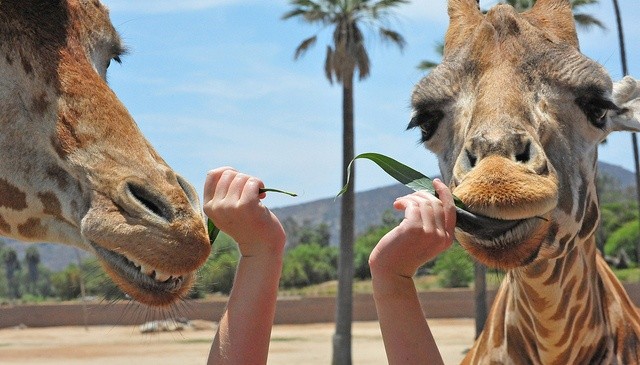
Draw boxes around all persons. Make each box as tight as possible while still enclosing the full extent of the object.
[203,166,456,365]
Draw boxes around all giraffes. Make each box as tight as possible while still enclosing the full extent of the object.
[0,0,235,338]
[405,0,640,364]
